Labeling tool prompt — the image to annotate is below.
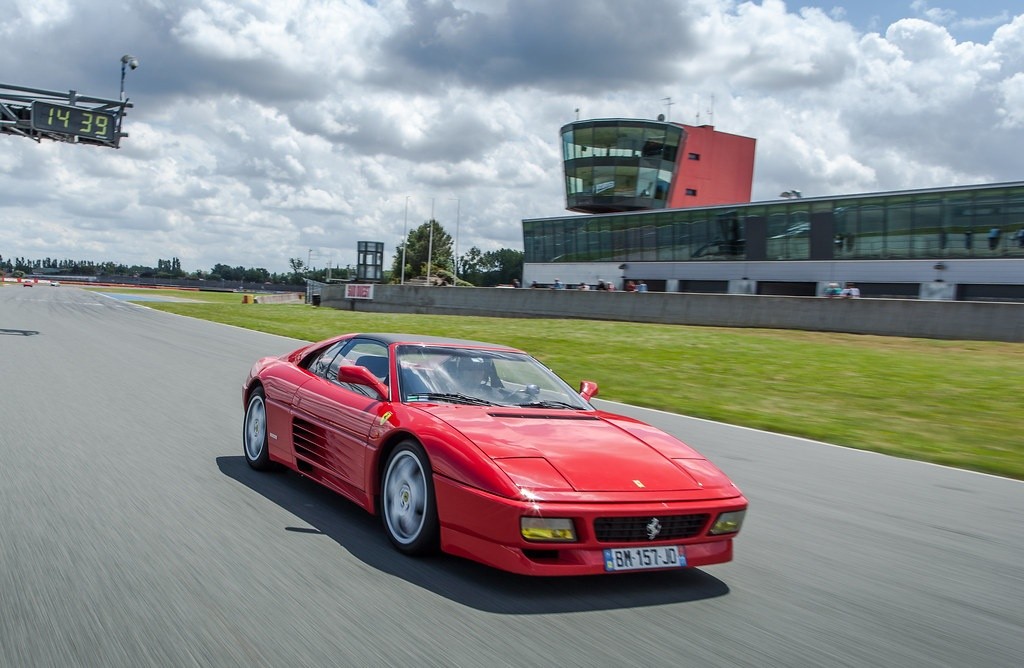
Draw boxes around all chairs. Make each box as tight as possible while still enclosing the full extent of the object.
[353,356,401,400]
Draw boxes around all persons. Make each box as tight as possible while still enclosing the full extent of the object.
[625,280,647,291]
[513,279,521,288]
[446,368,504,401]
[529,281,539,288]
[596,282,617,291]
[551,279,563,288]
[576,282,590,288]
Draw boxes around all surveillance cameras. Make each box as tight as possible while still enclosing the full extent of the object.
[130,60,139,69]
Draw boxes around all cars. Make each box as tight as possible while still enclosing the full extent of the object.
[51,280,60,286]
[23,280,32,287]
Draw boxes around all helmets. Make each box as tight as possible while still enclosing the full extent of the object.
[457,357,492,373]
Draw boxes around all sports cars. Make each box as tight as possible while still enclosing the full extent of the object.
[241,330,749,579]
